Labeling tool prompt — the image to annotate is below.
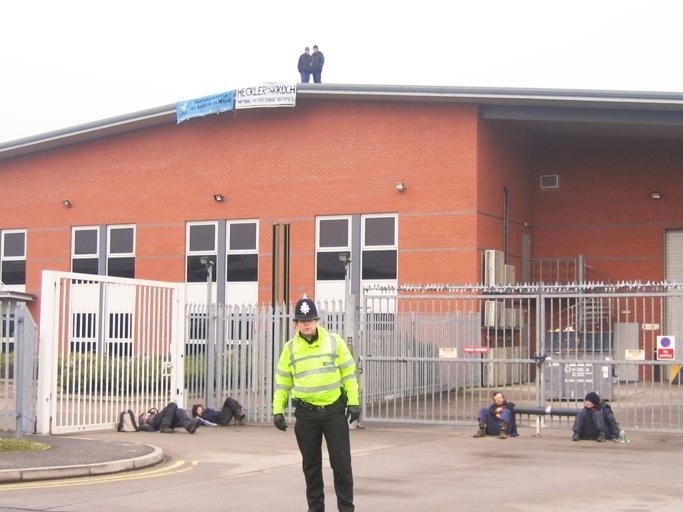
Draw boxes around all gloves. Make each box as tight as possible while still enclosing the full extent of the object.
[345,404,361,424]
[273,413,288,432]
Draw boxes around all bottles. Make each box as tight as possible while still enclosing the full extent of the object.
[620,430,627,442]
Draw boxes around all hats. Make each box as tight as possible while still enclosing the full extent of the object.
[305,45,319,51]
[585,392,599,405]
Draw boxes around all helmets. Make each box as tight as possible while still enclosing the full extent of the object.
[292,299,320,321]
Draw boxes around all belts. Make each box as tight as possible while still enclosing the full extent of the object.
[295,394,343,414]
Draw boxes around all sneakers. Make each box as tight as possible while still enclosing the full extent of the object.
[596,430,606,442]
[473,430,486,438]
[499,430,507,439]
[572,430,580,441]
[159,426,175,433]
[233,408,245,427]
[188,418,201,433]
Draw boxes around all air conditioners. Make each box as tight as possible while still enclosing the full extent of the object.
[481,248,529,388]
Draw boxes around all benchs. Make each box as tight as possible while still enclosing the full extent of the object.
[513,402,581,437]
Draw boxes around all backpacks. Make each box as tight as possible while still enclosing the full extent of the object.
[117,410,139,432]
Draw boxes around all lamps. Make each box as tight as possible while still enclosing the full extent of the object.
[395,181,407,193]
[214,195,225,202]
[648,191,664,201]
[63,200,71,208]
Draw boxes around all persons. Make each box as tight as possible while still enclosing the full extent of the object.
[139,401,200,434]
[310,45,324,83]
[298,46,312,83]
[470,391,519,438]
[271,300,361,512]
[572,392,620,443]
[192,396,245,427]
